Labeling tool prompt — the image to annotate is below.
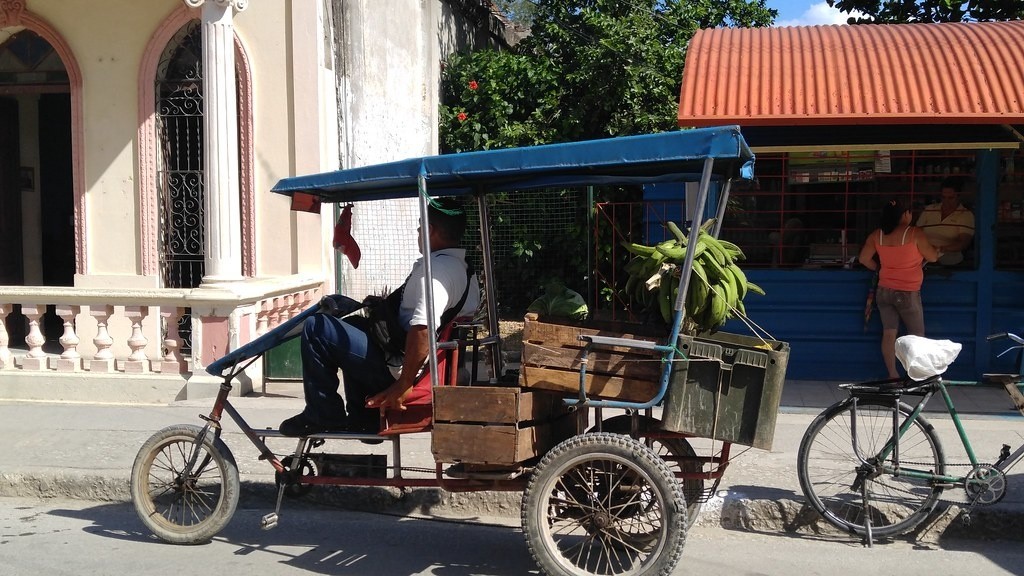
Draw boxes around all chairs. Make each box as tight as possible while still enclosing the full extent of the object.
[368,316,476,427]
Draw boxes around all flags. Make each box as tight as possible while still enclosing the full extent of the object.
[333,204,360,269]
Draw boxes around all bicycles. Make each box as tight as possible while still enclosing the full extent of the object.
[794,330,1024,546]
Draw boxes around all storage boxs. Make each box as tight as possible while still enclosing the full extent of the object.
[517,310,670,404]
[660,323,790,451]
[429,378,578,465]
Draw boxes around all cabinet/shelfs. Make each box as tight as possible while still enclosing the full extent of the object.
[892,154,1024,271]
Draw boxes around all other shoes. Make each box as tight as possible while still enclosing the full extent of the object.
[346,416,385,445]
[279,411,346,437]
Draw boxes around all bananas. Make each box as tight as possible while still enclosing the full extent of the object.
[619,216,766,334]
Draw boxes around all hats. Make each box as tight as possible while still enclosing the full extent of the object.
[332,204,361,269]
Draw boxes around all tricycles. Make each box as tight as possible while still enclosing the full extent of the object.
[128,123,788,576]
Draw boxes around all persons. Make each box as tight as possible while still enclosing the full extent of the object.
[916,186,976,265]
[860,196,938,387]
[523,258,590,453]
[280,198,480,444]
